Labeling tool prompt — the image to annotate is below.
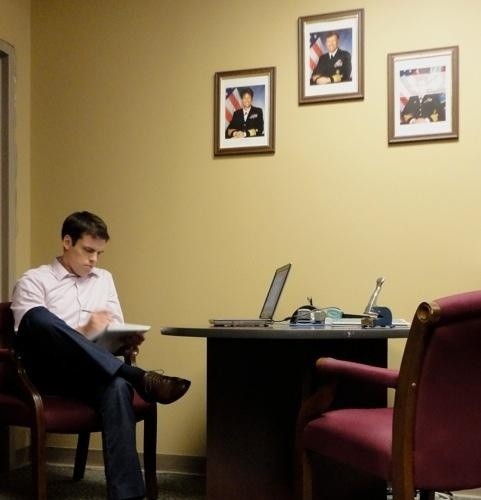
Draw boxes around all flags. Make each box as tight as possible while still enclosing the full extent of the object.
[224,87,246,132]
[308,31,327,79]
[400,64,445,112]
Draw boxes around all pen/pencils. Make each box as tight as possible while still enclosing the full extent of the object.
[82,309,95,314]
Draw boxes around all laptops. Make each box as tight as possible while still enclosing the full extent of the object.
[209,263,292,326]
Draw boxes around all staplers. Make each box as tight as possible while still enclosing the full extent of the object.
[370,306,392,327]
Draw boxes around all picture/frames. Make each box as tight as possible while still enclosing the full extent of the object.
[214,67,276,156]
[387,46,458,143]
[298,8,364,104]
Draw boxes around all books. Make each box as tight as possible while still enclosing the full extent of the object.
[95,320,152,356]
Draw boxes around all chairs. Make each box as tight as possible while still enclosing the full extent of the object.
[292,289,480,500]
[0,300,159,499]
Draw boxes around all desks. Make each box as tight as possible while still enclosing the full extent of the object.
[160,324,411,500]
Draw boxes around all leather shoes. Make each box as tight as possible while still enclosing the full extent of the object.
[134,368,191,405]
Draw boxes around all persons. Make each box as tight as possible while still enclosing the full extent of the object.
[9,211,193,500]
[402,78,446,125]
[311,30,353,86]
[225,87,265,138]
